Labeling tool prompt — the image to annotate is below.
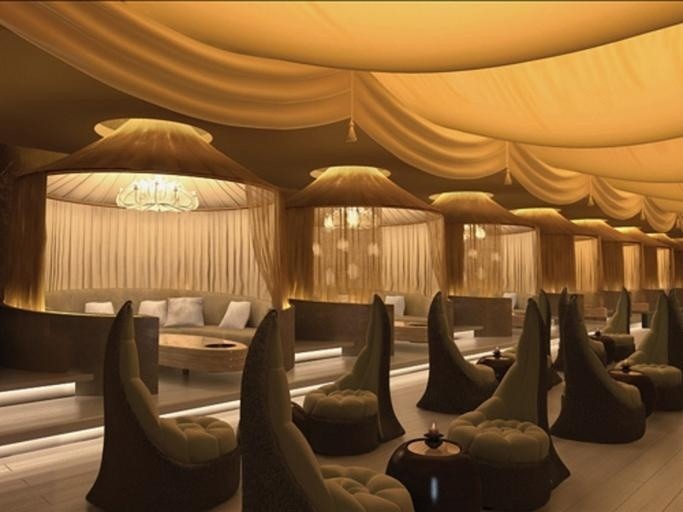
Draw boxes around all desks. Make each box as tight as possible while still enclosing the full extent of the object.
[386,437,480,510]
[283,285,683,366]
[608,367,653,418]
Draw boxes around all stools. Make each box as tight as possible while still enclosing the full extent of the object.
[633,362,682,411]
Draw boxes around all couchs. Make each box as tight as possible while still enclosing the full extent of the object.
[2,299,161,396]
[45,288,281,367]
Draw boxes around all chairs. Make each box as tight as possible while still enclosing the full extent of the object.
[448,298,570,510]
[552,296,647,443]
[87,299,240,510]
[416,290,497,415]
[301,293,406,458]
[237,311,414,510]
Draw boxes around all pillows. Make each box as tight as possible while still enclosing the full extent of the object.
[165,298,205,327]
[86,300,117,312]
[137,299,166,324]
[218,300,253,327]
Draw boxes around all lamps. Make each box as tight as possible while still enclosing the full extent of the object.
[513,207,595,241]
[17,119,281,215]
[645,233,682,249]
[571,220,640,246]
[426,191,535,239]
[615,226,671,248]
[284,165,443,230]
